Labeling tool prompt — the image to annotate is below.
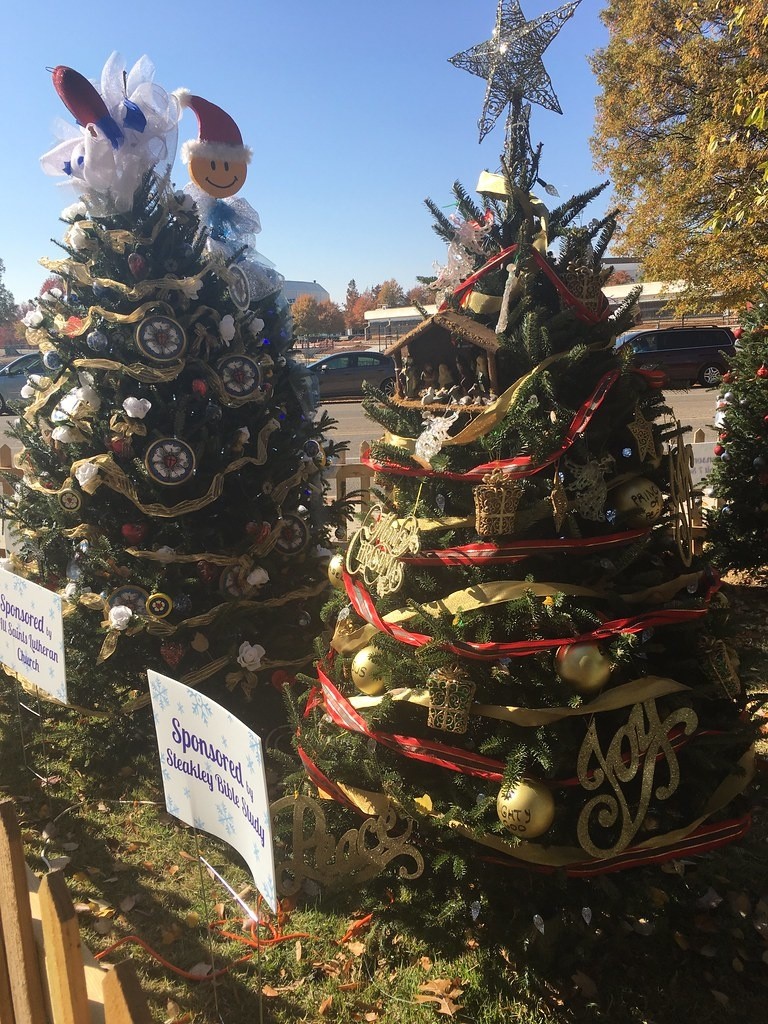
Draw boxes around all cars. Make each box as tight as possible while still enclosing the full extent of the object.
[0,351,55,417]
[305,351,403,400]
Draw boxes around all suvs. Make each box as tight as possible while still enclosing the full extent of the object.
[612,323,737,387]
